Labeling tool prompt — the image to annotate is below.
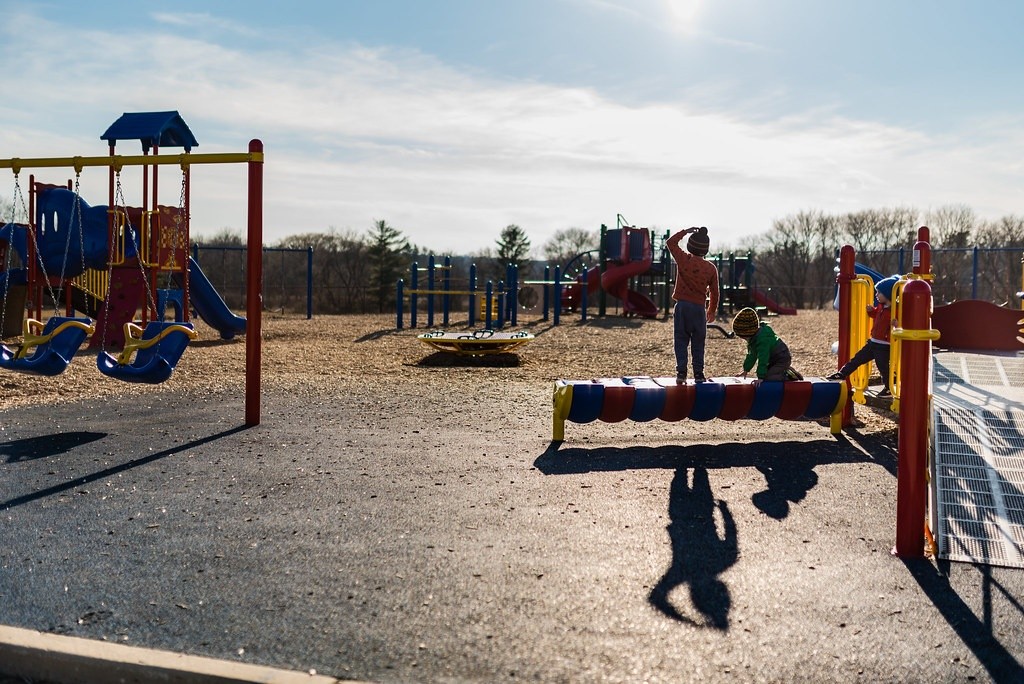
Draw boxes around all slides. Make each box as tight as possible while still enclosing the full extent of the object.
[188,255,247,340]
[561,260,616,311]
[600,260,658,319]
[752,287,797,316]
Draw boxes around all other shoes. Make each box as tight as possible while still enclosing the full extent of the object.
[785,366,804,381]
[676,373,687,384]
[694,373,706,383]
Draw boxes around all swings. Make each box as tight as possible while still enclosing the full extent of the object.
[223,249,245,311]
[96,170,198,385]
[263,250,284,313]
[0,174,96,377]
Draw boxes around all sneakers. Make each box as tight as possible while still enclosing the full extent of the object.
[876,387,893,398]
[826,373,847,382]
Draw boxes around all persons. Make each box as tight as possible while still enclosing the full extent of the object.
[666,227,720,383]
[826,274,900,398]
[732,307,804,387]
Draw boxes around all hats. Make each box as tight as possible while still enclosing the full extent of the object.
[733,307,760,337]
[874,274,903,301]
[687,227,709,257]
